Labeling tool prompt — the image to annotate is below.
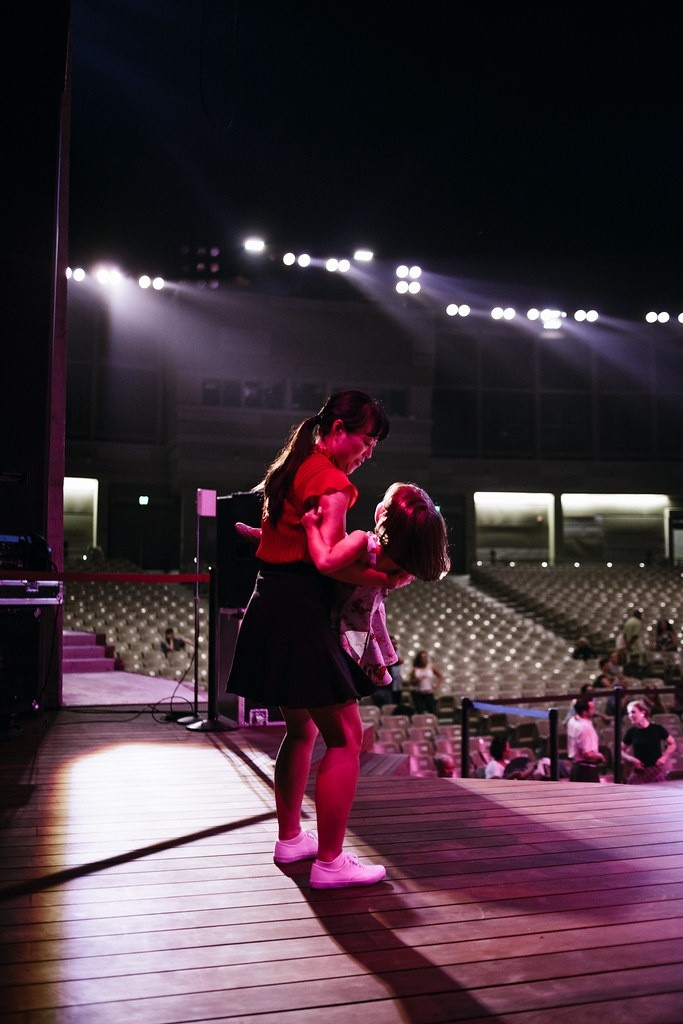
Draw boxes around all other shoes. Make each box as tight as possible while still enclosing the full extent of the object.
[235,522,261,543]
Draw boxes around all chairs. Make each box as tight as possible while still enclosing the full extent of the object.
[62,557,683,772]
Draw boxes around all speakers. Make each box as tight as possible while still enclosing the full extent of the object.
[208,491,266,610]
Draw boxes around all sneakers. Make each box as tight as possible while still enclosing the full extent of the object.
[273,831,318,863]
[308,852,387,889]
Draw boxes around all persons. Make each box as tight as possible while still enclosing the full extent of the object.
[225,388,390,888]
[390,634,445,717]
[558,609,683,783]
[235,482,451,686]
[161,628,185,658]
[434,734,551,780]
